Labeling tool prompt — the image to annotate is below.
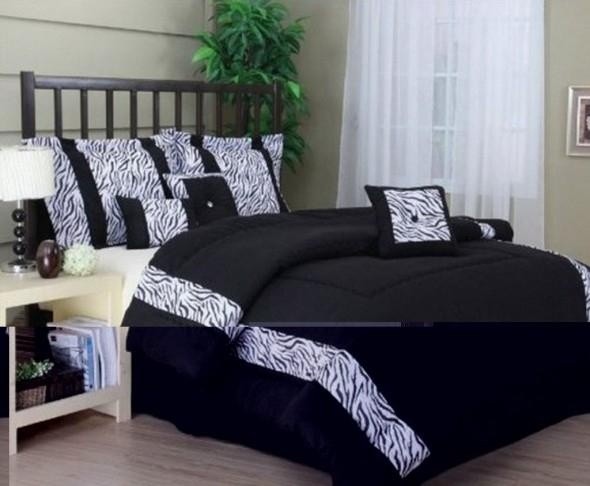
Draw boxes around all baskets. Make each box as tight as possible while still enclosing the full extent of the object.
[16,376,47,409]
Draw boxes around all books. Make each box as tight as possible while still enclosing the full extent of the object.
[45,315,118,391]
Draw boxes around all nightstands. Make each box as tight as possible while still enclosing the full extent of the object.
[1,270,132,456]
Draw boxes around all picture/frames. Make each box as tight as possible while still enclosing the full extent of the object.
[565,86,590,157]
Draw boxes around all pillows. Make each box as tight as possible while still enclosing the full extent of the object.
[366,184,462,257]
[20,130,291,249]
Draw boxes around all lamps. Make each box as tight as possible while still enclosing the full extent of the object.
[1,143,54,273]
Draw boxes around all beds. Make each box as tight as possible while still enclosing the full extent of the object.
[20,70,588,486]
